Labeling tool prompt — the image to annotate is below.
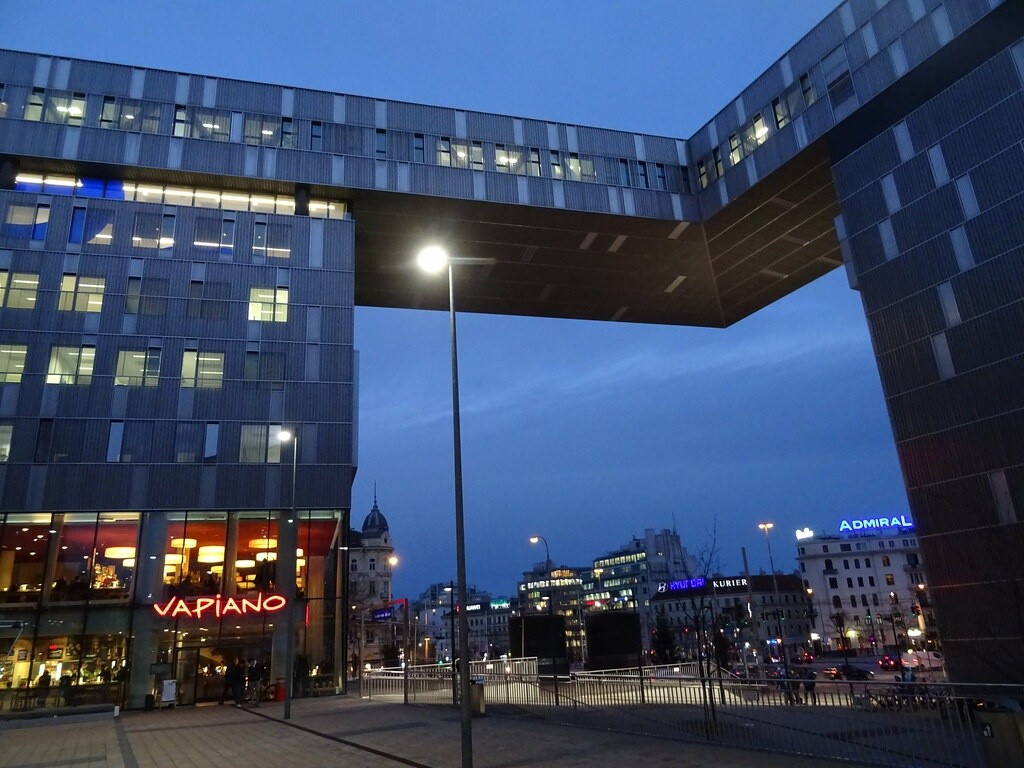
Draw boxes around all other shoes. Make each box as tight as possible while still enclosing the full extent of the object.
[236,703,241,708]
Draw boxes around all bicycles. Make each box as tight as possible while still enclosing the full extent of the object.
[871,686,941,712]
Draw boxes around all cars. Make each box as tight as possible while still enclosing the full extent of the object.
[731,653,818,683]
[879,656,896,670]
[823,663,875,681]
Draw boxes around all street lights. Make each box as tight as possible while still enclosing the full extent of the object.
[387,556,399,602]
[277,428,299,719]
[702,605,726,705]
[594,569,604,611]
[889,591,906,682]
[443,580,459,706]
[807,588,827,634]
[757,522,790,672]
[417,242,493,768]
[352,606,364,698]
[529,536,562,706]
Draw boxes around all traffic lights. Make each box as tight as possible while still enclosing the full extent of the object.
[911,607,916,616]
[867,609,871,618]
[915,606,921,615]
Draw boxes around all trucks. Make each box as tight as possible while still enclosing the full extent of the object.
[901,650,947,671]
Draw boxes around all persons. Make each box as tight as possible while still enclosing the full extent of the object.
[204,573,217,592]
[108,574,116,584]
[37,670,51,708]
[902,668,917,703]
[70,576,83,601]
[103,669,111,682]
[218,657,244,706]
[54,575,67,589]
[103,574,110,586]
[802,665,816,706]
[183,576,194,585]
[776,671,794,704]
[246,659,263,705]
[791,670,802,704]
[96,665,105,678]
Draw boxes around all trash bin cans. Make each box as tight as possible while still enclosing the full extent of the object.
[470,679,486,717]
[972,695,1024,768]
[275,677,286,702]
